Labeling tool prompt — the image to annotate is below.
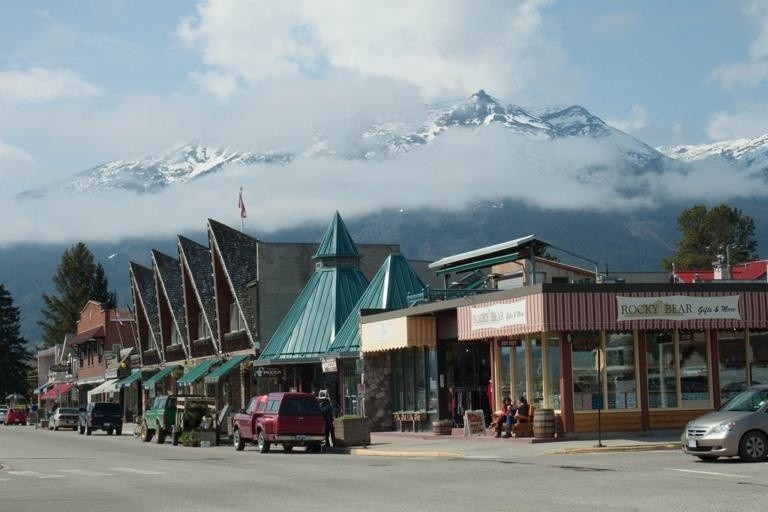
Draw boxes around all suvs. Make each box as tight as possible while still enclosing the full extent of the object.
[79,401,123,435]
[232,391,326,453]
[140,395,205,445]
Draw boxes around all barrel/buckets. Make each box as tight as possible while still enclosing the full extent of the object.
[534,409,556,437]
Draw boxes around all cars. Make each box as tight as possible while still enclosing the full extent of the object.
[49,403,79,435]
[681,384,768,462]
[0,405,27,425]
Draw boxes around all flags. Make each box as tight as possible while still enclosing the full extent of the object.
[238,191,247,218]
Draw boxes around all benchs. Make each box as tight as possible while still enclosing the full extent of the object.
[491,403,535,437]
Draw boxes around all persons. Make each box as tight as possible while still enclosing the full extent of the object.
[502,396,527,437]
[320,397,336,446]
[494,396,514,438]
